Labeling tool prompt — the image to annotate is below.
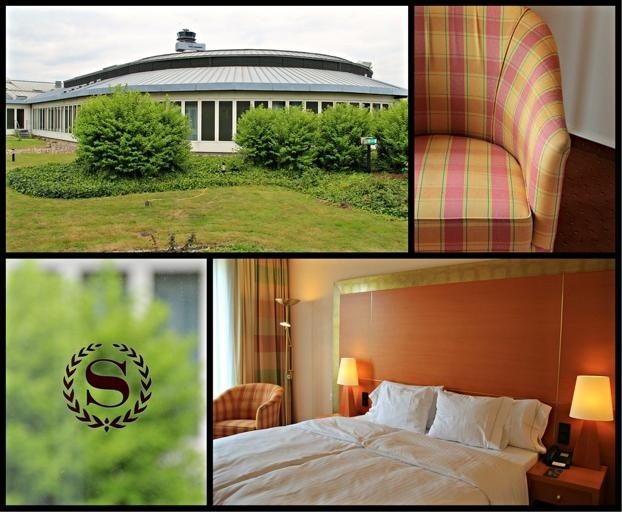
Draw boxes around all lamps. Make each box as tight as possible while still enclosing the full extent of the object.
[335,356,358,416]
[273,296,300,424]
[567,375,615,472]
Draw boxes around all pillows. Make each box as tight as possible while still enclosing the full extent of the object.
[363,379,552,457]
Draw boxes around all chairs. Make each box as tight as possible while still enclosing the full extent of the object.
[414,4,574,252]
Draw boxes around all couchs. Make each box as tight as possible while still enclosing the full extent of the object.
[211,382,286,438]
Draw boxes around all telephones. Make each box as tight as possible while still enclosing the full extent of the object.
[545,445,571,469]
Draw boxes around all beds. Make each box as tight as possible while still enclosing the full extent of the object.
[210,380,554,506]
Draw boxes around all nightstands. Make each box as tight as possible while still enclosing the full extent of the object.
[527,460,608,510]
